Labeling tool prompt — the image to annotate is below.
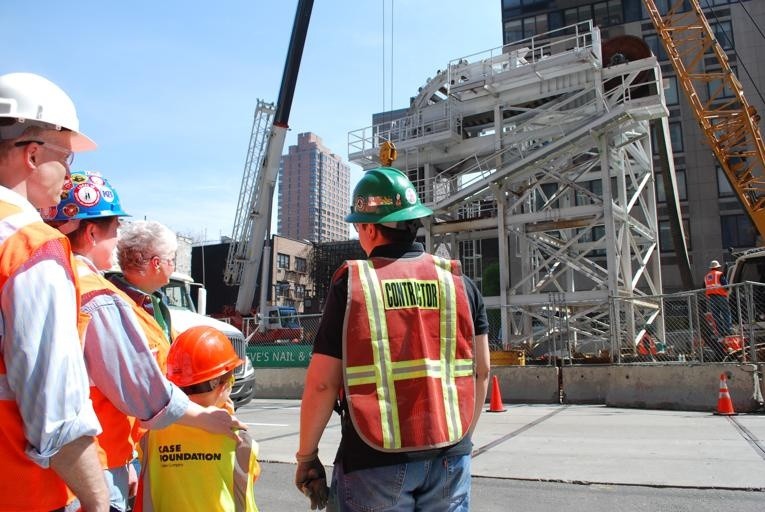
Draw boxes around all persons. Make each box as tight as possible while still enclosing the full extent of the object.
[701,258,736,334]
[1,71,111,512]
[636,324,662,359]
[135,327,260,511]
[293,165,494,512]
[719,327,743,355]
[33,167,247,512]
[101,222,182,511]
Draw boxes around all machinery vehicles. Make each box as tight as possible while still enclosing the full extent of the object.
[210,1,396,342]
[639,0,765,364]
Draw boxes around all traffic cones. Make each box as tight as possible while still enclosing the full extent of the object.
[484,375,507,413]
[713,376,738,416]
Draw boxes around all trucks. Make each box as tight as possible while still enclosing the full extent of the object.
[149,269,255,411]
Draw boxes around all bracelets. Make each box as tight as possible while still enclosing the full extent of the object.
[296,449,320,462]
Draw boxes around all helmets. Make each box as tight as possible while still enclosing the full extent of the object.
[344,167,433,224]
[166,326,245,388]
[36,170,133,221]
[0,72,97,152]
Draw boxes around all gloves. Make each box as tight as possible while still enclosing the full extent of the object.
[296,449,330,511]
[302,478,328,510]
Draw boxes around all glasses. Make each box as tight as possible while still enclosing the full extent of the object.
[14,140,74,166]
[143,257,178,264]
